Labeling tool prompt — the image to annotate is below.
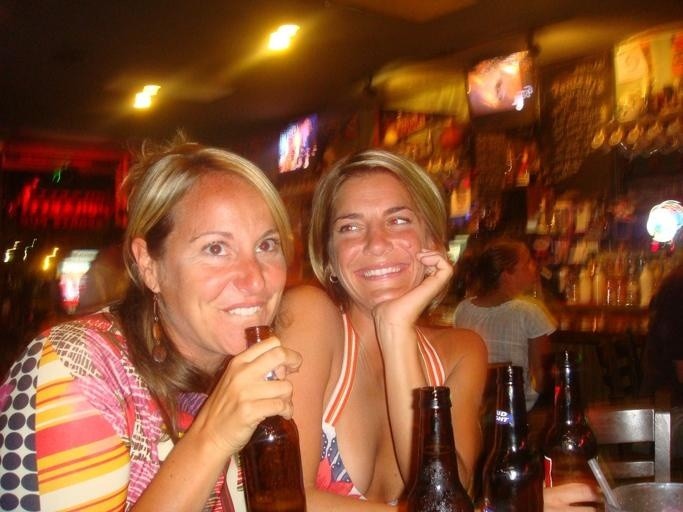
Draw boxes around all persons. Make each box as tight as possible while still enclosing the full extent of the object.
[639,267,682,471]
[273,148,490,511]
[0,131,303,511]
[1,222,130,385]
[447,236,556,415]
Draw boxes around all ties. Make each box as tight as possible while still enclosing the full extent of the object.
[274,112,322,174]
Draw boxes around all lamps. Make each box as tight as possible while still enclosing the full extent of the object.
[527,408,671,485]
[594,328,643,404]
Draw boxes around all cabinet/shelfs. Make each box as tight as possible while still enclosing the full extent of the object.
[405,385,476,512]
[236,324,308,512]
[531,196,608,258]
[539,347,600,512]
[391,107,462,175]
[500,137,542,186]
[566,249,675,309]
[481,363,546,512]
